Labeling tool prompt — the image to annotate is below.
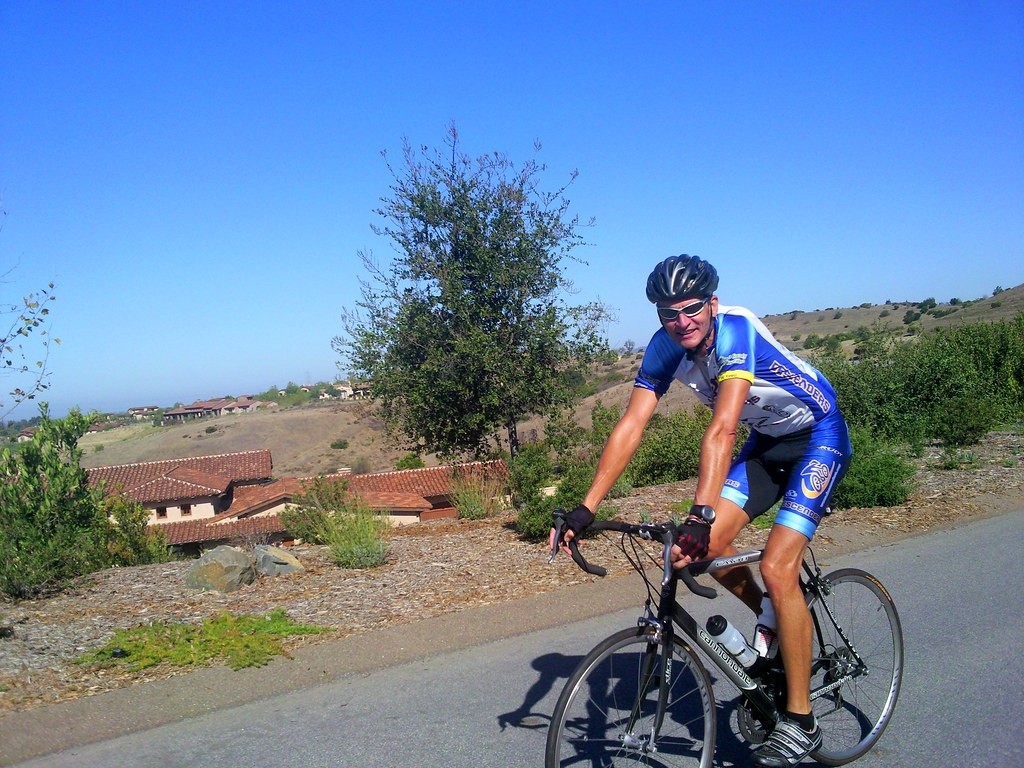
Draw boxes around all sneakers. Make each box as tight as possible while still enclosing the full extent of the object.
[749,713,823,768]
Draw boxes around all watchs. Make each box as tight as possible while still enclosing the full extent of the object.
[690,504,716,525]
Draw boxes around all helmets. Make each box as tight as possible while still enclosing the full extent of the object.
[646,254,719,304]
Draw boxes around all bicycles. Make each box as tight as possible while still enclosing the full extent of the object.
[544,465,904,768]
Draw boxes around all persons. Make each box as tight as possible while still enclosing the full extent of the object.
[550,255,852,768]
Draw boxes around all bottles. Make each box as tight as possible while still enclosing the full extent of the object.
[705,614,758,668]
[753,591,779,659]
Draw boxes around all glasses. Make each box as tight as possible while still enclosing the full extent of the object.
[656,297,708,320]
